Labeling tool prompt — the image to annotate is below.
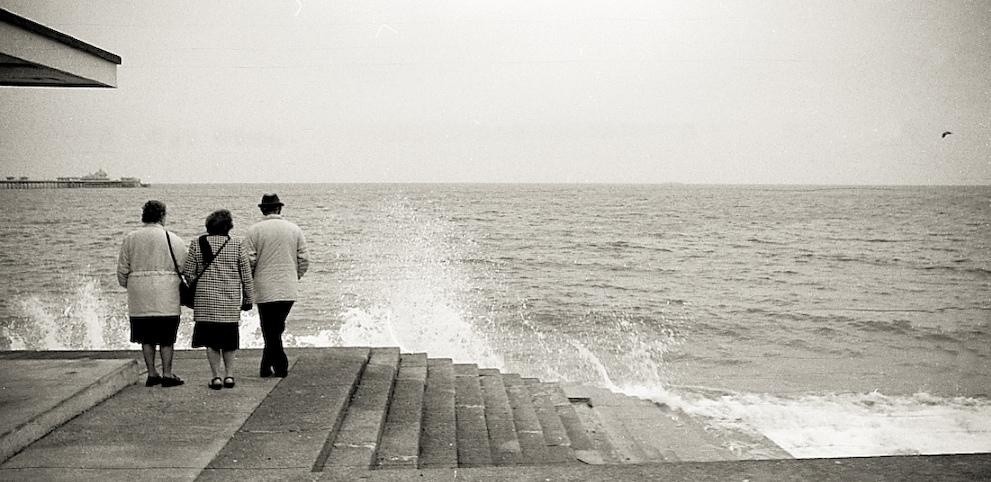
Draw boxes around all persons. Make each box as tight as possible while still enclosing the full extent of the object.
[242,193,310,378]
[184,208,254,390]
[117,200,189,387]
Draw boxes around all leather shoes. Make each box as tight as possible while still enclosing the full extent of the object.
[146,374,184,387]
[260,368,288,377]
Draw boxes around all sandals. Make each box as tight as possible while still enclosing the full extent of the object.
[208,376,235,390]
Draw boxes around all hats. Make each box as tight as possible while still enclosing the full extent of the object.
[258,193,284,207]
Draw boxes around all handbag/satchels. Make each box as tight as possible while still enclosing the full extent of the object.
[179,279,197,309]
